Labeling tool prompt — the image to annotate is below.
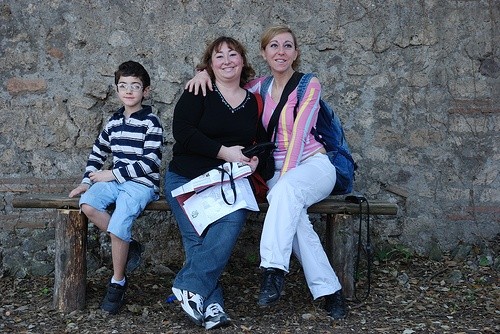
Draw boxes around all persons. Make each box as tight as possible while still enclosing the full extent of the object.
[185,26,348,321]
[69,59,163,314]
[163,36,271,331]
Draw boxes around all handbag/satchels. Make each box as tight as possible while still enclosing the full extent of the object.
[250,173,269,204]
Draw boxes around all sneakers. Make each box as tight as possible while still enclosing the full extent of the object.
[201,303,231,330]
[325,288,347,318]
[103,272,128,313]
[125,238,145,272]
[257,268,284,306]
[172,287,205,325]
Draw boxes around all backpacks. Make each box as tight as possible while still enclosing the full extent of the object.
[260,72,358,196]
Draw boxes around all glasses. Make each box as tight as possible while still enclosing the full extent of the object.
[117,83,145,91]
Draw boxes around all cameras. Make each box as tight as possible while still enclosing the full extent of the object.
[345,194,364,204]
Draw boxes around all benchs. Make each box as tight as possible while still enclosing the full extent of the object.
[12,187,398,314]
